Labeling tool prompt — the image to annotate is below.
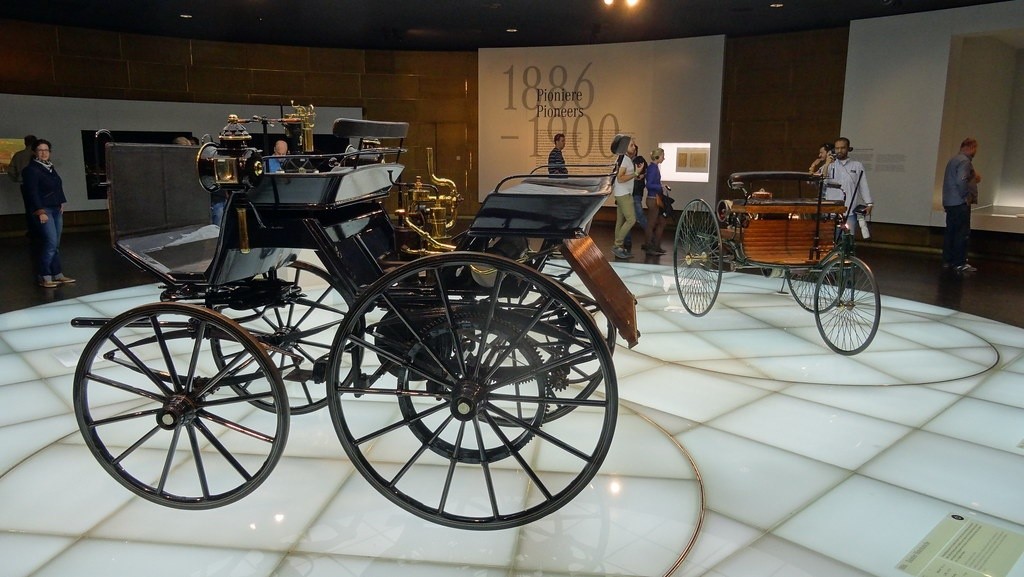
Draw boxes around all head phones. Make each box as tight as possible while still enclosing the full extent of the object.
[655,156,659,159]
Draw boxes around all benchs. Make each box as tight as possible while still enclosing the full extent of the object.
[443,134,633,297]
[235,116,410,245]
[722,171,857,269]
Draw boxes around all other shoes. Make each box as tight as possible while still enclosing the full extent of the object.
[654,244,667,253]
[641,242,648,250]
[39,281,57,287]
[624,252,633,257]
[953,264,977,272]
[611,247,627,259]
[54,277,76,283]
[645,250,661,256]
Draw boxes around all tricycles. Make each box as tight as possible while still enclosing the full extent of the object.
[672,169,882,359]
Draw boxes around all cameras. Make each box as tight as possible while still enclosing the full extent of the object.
[830,154,836,158]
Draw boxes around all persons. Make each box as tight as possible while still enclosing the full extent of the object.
[942,137,981,273]
[23,139,76,287]
[175,137,288,227]
[610,137,669,258]
[548,134,568,177]
[8,136,38,239]
[809,137,874,287]
